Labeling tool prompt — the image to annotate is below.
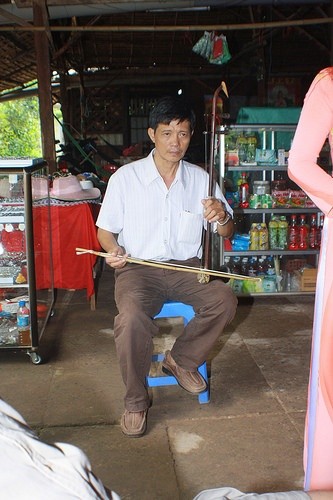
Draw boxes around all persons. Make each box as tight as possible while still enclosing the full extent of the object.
[286,64,333,491]
[0,398,333,500]
[95,95,239,436]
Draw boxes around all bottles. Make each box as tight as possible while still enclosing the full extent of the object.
[250,223,259,250]
[289,215,298,250]
[253,180,307,199]
[309,215,317,250]
[240,173,247,208]
[317,215,324,249]
[17,301,31,345]
[259,223,268,251]
[298,215,309,250]
[278,216,289,250]
[269,216,278,250]
[224,256,276,277]
[236,131,257,164]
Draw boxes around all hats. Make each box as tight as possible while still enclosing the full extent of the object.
[48,175,102,200]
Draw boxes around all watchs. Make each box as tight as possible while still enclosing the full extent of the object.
[216,211,232,226]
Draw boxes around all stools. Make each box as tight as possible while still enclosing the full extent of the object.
[143,300,212,404]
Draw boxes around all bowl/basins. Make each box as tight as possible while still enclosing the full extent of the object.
[80,181,93,189]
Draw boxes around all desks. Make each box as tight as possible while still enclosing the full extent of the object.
[53,188,105,310]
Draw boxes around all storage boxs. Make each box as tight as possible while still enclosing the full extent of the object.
[291,268,318,291]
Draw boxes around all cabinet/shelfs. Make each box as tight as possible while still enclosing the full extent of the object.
[0,160,56,365]
[212,124,333,296]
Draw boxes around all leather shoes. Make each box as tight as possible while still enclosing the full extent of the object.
[163,350,207,393]
[120,408,147,437]
